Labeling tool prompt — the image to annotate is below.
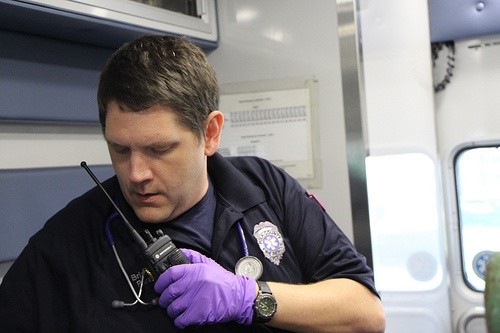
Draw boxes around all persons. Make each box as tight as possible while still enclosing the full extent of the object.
[1,35,387,333]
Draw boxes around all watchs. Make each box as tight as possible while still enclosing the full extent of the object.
[250,281,277,328]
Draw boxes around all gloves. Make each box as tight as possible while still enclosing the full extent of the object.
[153,246,257,329]
